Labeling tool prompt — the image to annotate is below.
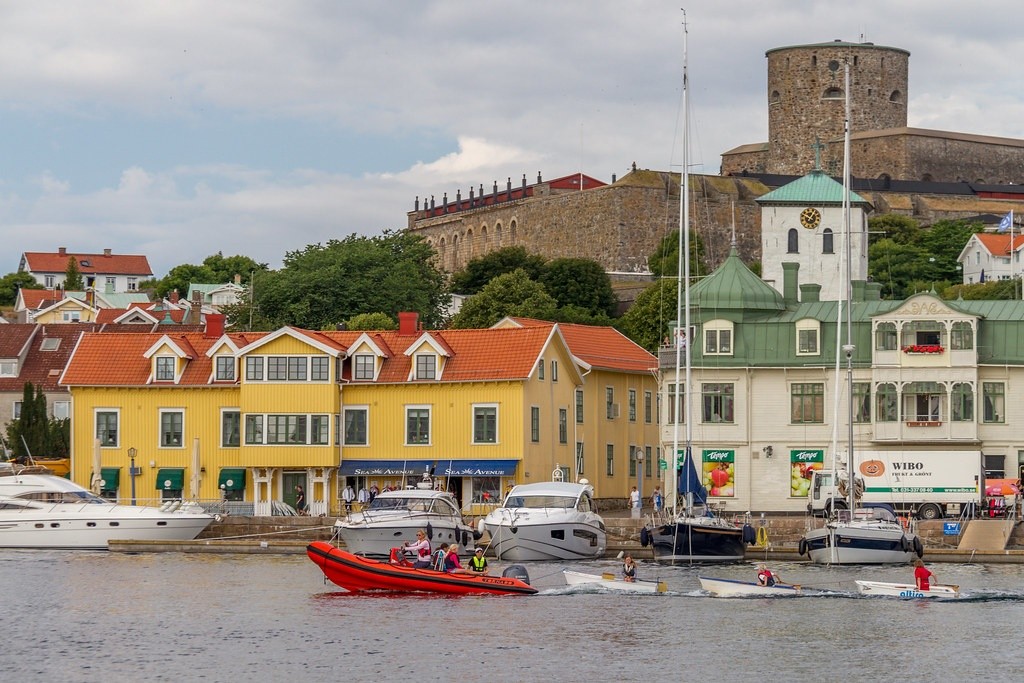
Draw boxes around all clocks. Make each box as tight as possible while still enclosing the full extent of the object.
[800,207,821,229]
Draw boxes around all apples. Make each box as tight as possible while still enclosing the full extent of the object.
[712,469,728,488]
[791,468,811,497]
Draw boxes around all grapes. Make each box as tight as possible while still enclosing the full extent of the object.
[702,463,734,491]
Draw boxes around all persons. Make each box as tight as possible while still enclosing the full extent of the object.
[757,564,784,587]
[432,543,448,568]
[402,529,432,568]
[649,485,664,511]
[466,547,490,576]
[622,555,637,582]
[296,486,308,516]
[914,559,938,592]
[444,544,465,572]
[654,491,661,511]
[628,486,639,509]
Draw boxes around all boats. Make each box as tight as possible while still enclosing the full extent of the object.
[697,575,803,599]
[563,570,666,596]
[862,580,960,601]
[339,462,485,561]
[305,538,542,602]
[482,481,607,563]
[0,432,229,549]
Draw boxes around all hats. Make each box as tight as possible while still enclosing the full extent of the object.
[754,564,765,570]
[475,548,482,553]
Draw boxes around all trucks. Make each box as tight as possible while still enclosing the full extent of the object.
[807,450,988,523]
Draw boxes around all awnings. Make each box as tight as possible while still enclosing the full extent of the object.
[90,468,119,490]
[336,459,519,477]
[155,469,184,490]
[217,469,246,489]
[63,471,70,480]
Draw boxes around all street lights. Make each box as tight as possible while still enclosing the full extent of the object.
[128,447,139,505]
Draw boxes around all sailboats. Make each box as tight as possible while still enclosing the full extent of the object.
[800,55,925,570]
[640,9,770,564]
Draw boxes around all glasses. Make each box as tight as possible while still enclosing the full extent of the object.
[416,534,422,536]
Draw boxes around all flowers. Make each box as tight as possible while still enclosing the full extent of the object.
[904,345,944,354]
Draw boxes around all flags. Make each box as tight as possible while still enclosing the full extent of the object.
[997,211,1012,232]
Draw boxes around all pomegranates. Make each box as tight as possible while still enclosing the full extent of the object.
[710,482,734,496]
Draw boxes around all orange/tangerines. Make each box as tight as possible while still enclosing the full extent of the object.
[703,462,720,472]
[812,462,823,469]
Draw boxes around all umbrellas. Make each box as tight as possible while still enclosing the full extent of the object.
[985,482,1021,495]
